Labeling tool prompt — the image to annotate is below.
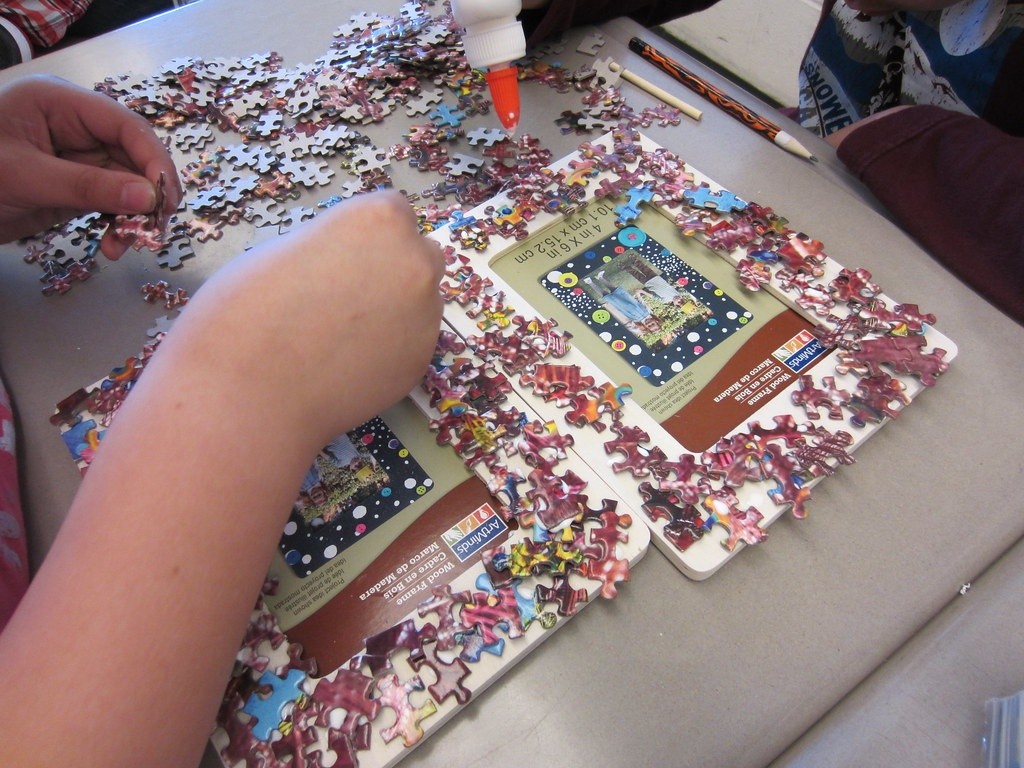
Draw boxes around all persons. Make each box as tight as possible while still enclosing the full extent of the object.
[490,0,1024,329]
[0,71,449,768]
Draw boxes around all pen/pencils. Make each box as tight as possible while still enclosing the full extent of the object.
[626,34,820,162]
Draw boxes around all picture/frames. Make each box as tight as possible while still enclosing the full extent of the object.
[428,121,958,584]
[54,320,651,768]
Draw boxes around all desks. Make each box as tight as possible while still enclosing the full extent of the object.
[0,0,1024,768]
[769,533,1024,768]
